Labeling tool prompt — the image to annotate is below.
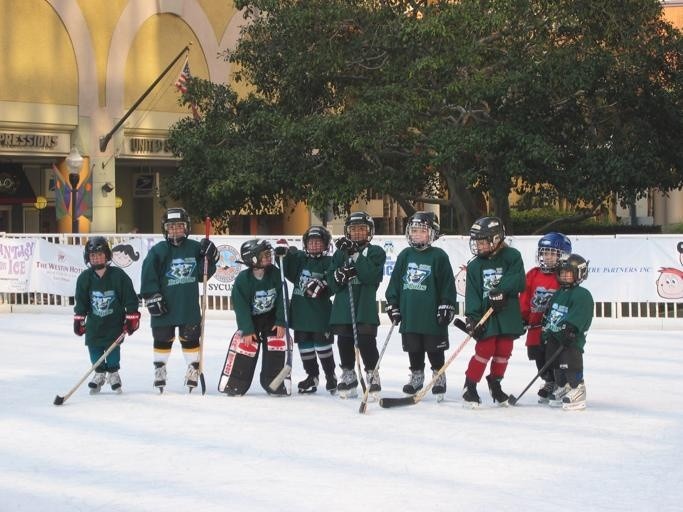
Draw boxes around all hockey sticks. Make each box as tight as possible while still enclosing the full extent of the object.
[360,321,395,414]
[270,255,291,393]
[345,251,368,396]
[198,217,208,396]
[508,344,561,403]
[378,307,495,408]
[54,329,127,404]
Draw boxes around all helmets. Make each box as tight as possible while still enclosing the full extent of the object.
[405,210,441,251]
[468,216,506,257]
[344,211,375,245]
[556,254,589,289]
[239,238,275,270]
[534,232,572,274]
[159,207,192,246]
[302,225,332,256]
[84,235,113,263]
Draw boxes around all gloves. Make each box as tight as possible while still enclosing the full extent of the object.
[122,312,141,337]
[302,278,328,300]
[73,313,87,336]
[198,238,220,266]
[466,317,488,341]
[333,262,359,284]
[562,323,577,344]
[436,304,456,327]
[489,288,508,314]
[335,236,357,256]
[520,319,528,336]
[145,292,169,321]
[384,304,402,326]
[275,238,289,259]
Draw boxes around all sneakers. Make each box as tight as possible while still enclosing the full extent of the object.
[337,367,358,392]
[431,371,447,395]
[402,366,425,394]
[489,381,508,402]
[297,373,320,390]
[185,363,200,388]
[537,382,586,405]
[325,371,337,391]
[108,368,122,391]
[87,368,106,389]
[460,381,480,403]
[154,361,167,388]
[365,368,381,392]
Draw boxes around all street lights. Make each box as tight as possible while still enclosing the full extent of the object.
[63,143,85,304]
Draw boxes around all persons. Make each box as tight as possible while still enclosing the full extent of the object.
[520,232,572,397]
[463,217,526,405]
[385,211,457,391]
[139,208,220,387]
[540,253,594,405]
[327,212,385,392]
[227,240,289,395]
[73,234,140,390]
[274,227,337,391]
